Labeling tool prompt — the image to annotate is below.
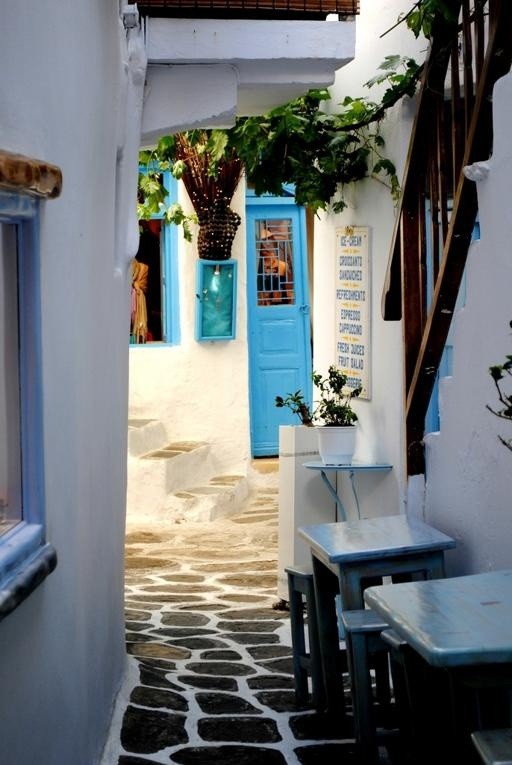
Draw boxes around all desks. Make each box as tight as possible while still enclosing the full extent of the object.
[363,569,512,668]
[305,462,393,522]
[298,518,456,755]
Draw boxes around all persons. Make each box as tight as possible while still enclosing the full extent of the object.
[258,229,291,304]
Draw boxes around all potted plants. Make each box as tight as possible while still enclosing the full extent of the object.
[310,367,367,465]
[137,2,442,264]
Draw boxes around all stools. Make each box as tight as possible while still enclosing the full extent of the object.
[381,627,410,707]
[471,730,511,764]
[340,607,388,761]
[284,566,314,710]
